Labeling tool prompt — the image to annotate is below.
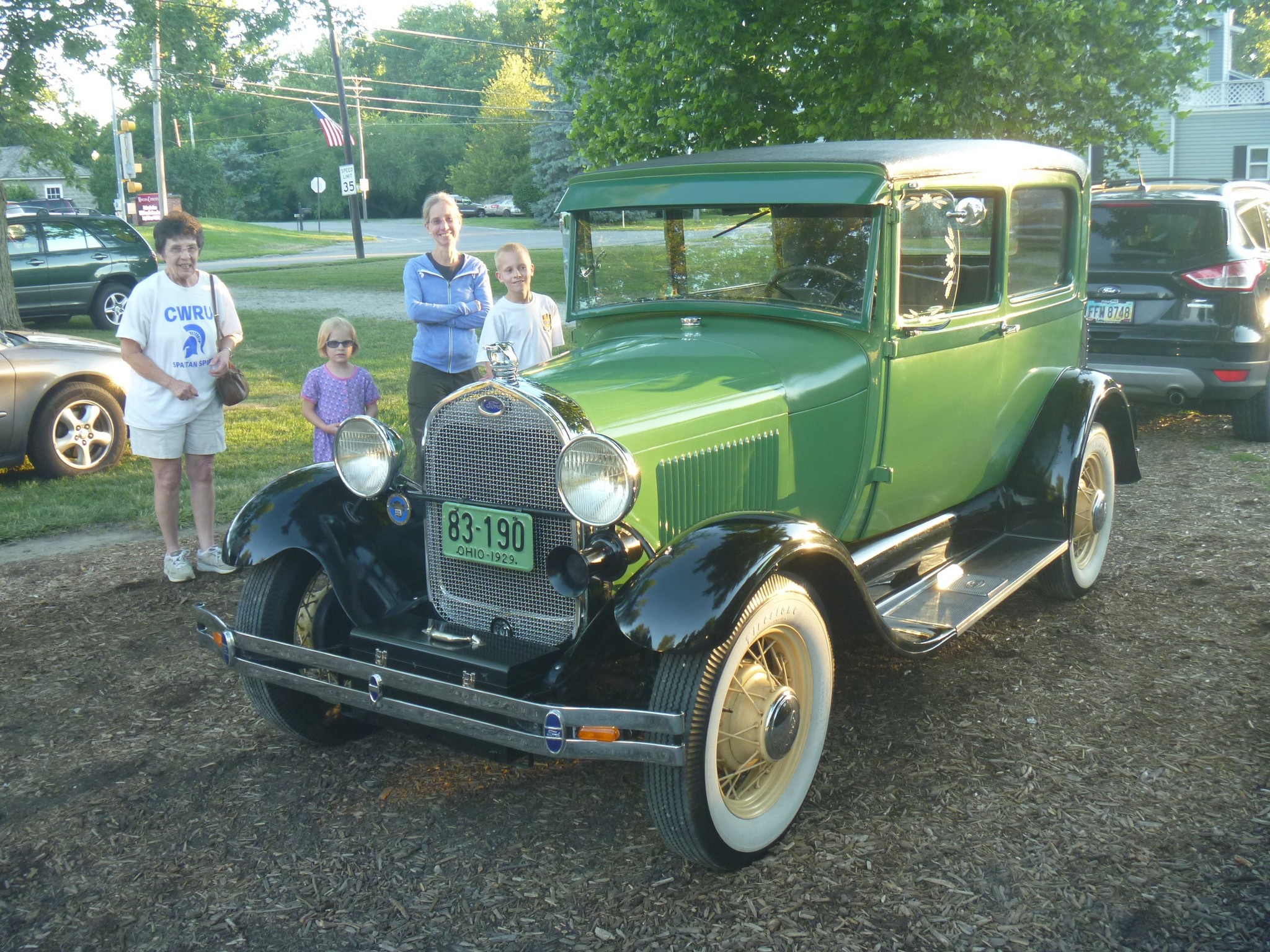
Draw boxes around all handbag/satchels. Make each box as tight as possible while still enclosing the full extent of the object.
[215,360,250,407]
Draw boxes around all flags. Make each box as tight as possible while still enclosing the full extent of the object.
[310,102,356,146]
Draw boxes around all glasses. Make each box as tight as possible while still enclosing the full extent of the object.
[161,244,202,255]
[324,339,356,348]
[427,214,461,225]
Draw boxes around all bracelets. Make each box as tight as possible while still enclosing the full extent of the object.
[226,347,232,357]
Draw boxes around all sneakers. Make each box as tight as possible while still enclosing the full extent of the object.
[163,548,196,582]
[196,543,239,574]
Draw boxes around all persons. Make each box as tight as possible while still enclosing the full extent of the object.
[475,242,565,382]
[300,317,380,463]
[116,210,243,583]
[404,191,493,486]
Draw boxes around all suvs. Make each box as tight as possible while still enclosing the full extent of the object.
[16,197,82,214]
[6,201,24,216]
[1085,177,1270,442]
[4,204,158,331]
[448,194,485,218]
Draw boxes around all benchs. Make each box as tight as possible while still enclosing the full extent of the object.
[895,267,993,307]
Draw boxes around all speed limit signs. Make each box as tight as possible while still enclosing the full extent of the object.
[339,164,357,196]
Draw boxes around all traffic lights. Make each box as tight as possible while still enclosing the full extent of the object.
[120,119,136,131]
[127,181,142,193]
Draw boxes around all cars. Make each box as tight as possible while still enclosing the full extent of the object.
[190,138,1142,872]
[483,199,526,217]
[0,318,132,480]
